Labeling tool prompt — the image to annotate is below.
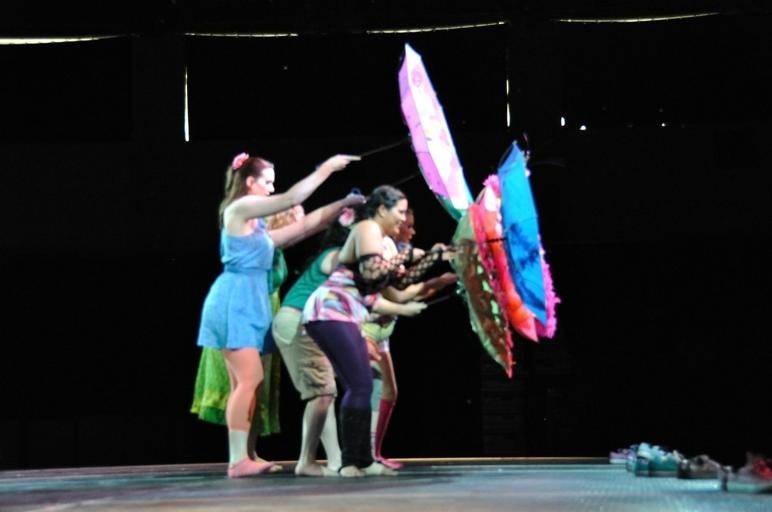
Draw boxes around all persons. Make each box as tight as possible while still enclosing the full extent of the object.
[195,152,364,479]
[191,194,369,478]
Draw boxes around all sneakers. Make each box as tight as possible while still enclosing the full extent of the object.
[609,442,772,494]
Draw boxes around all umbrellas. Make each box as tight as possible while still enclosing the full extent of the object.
[453,140,561,375]
[363,42,463,202]
